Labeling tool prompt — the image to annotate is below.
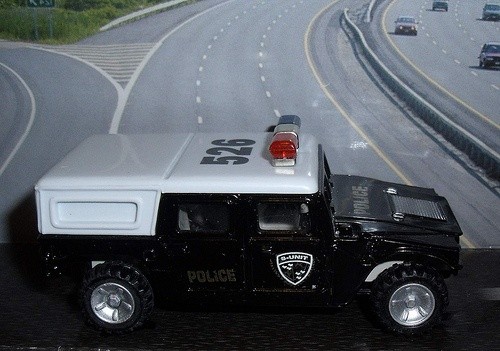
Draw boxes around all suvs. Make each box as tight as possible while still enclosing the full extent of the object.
[35,114,461,341]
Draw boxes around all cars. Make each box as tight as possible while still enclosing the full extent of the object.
[394,1,500,69]
